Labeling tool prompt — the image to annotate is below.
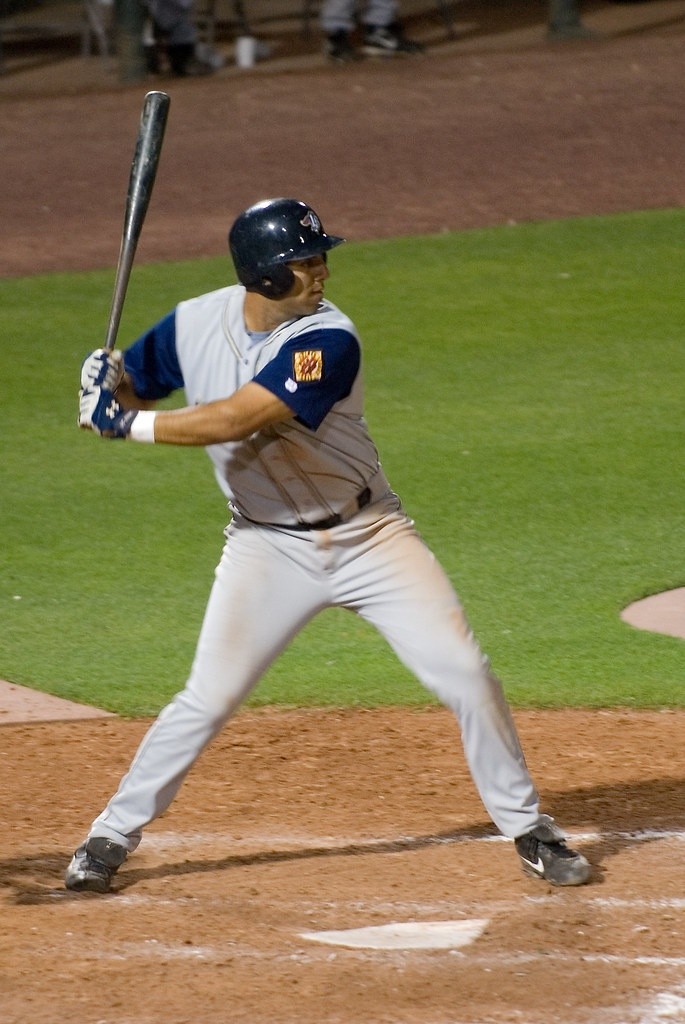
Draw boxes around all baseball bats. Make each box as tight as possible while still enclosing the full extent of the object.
[102,86,174,357]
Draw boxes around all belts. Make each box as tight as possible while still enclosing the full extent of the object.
[237,486,372,532]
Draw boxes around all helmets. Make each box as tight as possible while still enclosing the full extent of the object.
[228,198,347,300]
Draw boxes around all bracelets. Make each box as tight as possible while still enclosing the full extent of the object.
[126,410,155,447]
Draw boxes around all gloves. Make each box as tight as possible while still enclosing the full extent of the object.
[81,347,124,394]
[77,385,138,441]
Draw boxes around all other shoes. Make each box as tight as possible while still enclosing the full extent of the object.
[361,22,425,57]
[327,33,363,60]
[145,40,213,79]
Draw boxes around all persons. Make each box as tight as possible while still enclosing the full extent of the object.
[65,196,590,893]
[99,0,595,77]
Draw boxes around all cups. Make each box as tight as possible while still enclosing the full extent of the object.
[234,37,255,68]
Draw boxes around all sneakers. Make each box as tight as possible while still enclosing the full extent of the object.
[65,838,127,893]
[514,824,591,886]
